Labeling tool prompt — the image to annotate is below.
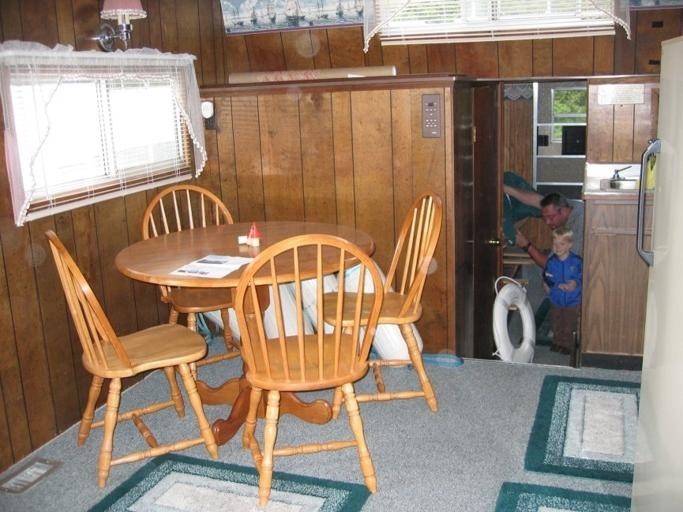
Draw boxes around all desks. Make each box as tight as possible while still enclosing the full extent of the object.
[113,222,375,444]
[502,244,536,283]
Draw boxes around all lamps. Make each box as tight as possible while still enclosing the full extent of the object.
[95,0,147,52]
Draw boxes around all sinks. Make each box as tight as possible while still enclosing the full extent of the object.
[600,179,637,191]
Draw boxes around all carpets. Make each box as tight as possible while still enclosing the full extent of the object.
[495,482,632,512]
[524,373,640,482]
[87,454,371,512]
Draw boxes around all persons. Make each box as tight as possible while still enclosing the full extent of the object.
[540,226,583,355]
[501,180,583,345]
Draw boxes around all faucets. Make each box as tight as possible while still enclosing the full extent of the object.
[611,165,632,183]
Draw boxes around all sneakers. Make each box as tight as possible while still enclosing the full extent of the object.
[548,342,572,356]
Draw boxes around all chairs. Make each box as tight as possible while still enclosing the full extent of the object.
[239,232,384,508]
[320,190,444,420]
[43,230,220,490]
[141,186,243,383]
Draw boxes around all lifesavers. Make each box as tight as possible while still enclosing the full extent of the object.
[492,282,538,364]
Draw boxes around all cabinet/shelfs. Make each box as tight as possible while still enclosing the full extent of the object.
[580,194,656,358]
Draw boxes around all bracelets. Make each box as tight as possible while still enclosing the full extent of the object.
[521,242,531,252]
